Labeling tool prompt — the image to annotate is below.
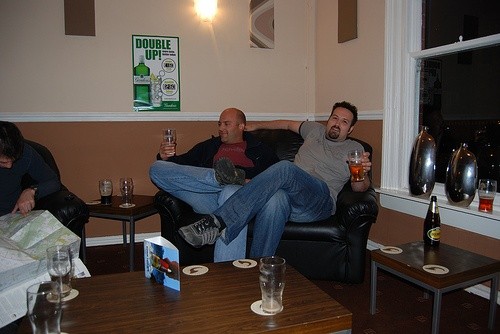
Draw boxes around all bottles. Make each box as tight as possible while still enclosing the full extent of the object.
[446,143,477,207]
[408,126,436,200]
[423,196,442,248]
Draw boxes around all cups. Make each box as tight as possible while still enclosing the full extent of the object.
[259,256,286,314]
[45,245,73,298]
[478,179,497,213]
[26,282,62,334]
[99,180,112,205]
[120,177,133,207]
[344,150,370,182]
[164,130,176,155]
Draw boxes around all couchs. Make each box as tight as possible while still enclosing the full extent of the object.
[151,129,379,283]
[26,139,93,234]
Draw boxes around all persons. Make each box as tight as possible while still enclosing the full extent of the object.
[150,107,269,264]
[178,100,372,259]
[0,120,61,216]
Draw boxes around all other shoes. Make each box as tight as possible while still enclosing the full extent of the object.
[215,157,245,184]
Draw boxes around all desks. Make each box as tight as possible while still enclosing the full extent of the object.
[370,239,500,334]
[80,195,159,272]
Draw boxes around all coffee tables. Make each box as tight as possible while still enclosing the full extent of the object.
[16,257,353,334]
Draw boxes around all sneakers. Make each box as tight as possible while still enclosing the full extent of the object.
[178,216,222,247]
